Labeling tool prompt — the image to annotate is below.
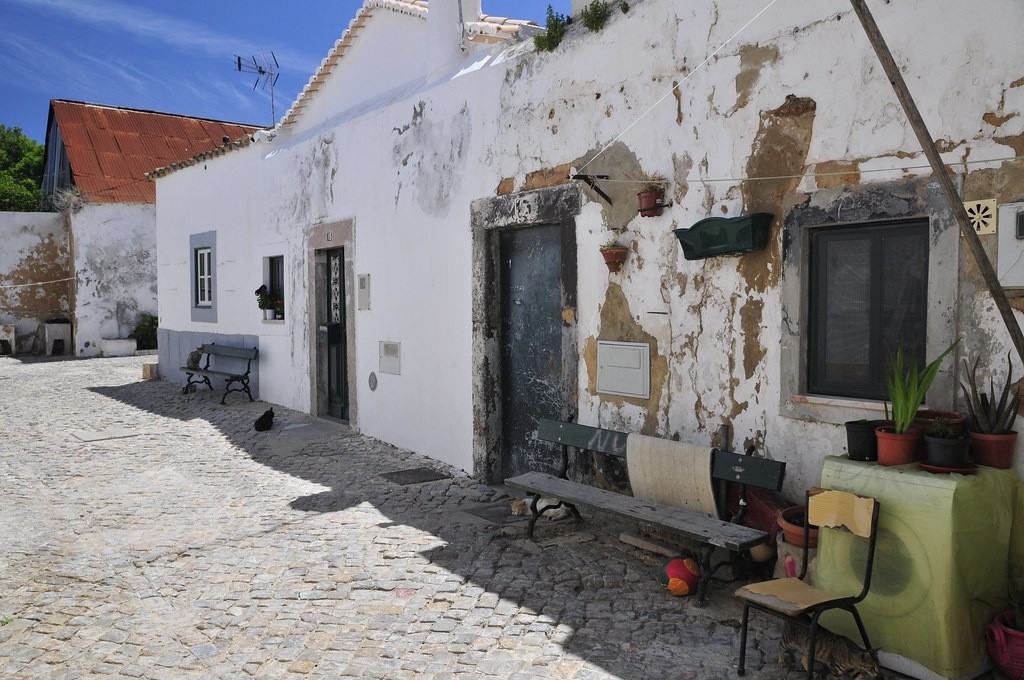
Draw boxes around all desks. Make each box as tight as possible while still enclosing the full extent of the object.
[812,454,1024,680]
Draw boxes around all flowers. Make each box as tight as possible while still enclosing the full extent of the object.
[274,301,283,315]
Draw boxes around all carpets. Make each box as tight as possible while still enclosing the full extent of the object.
[461,499,531,525]
[378,467,450,486]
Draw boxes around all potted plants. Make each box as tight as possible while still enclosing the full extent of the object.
[969,566,1024,680]
[875,335,963,466]
[845,419,878,462]
[254,284,278,320]
[951,348,1020,469]
[599,240,627,272]
[623,169,670,217]
[924,413,970,468]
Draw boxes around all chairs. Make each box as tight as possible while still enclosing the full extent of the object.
[735,486,881,680]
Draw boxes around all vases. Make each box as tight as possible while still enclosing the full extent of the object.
[275,315,282,319]
[101,338,136,356]
[912,409,966,435]
[672,212,775,261]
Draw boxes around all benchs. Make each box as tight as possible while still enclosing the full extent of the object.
[504,419,786,607]
[178,343,258,404]
